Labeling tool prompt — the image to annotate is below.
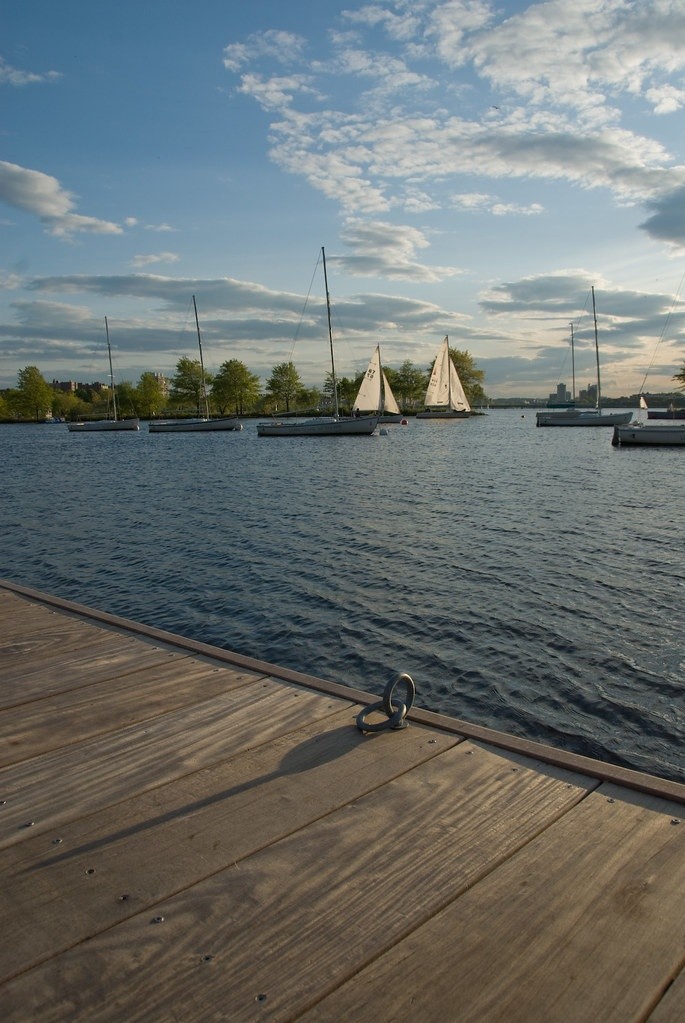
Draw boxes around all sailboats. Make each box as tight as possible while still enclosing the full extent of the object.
[256,245,386,438]
[149,294,245,431]
[413,334,472,419]
[65,314,141,432]
[610,269,685,448]
[533,285,634,429]
[349,341,408,425]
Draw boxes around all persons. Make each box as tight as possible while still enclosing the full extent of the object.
[356,408,361,418]
[426,406,431,413]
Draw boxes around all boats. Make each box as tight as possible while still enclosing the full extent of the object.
[647,408,685,420]
